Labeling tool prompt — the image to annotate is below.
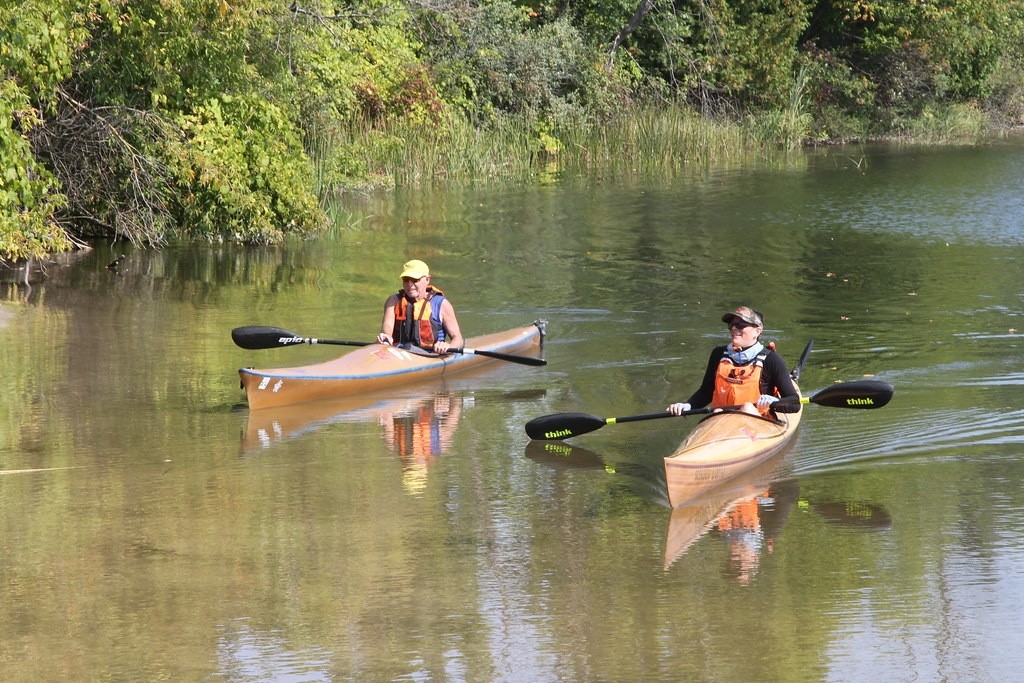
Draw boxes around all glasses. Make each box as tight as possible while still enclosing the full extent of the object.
[726,320,755,329]
[403,277,420,283]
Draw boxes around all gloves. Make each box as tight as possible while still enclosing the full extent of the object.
[757,394,779,412]
[666,403,691,416]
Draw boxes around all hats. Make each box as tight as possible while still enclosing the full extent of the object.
[722,305,763,326]
[399,259,429,279]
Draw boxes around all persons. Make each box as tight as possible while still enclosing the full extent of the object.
[666,307,801,418]
[376,259,465,358]
[710,475,791,586]
[379,395,462,496]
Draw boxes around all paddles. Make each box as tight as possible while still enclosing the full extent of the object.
[525,380,895,441]
[524,439,892,529]
[231,325,547,366]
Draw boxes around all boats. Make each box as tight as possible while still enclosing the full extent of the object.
[238,345,545,460]
[239,318,549,411]
[664,425,801,572]
[664,339,815,509]
